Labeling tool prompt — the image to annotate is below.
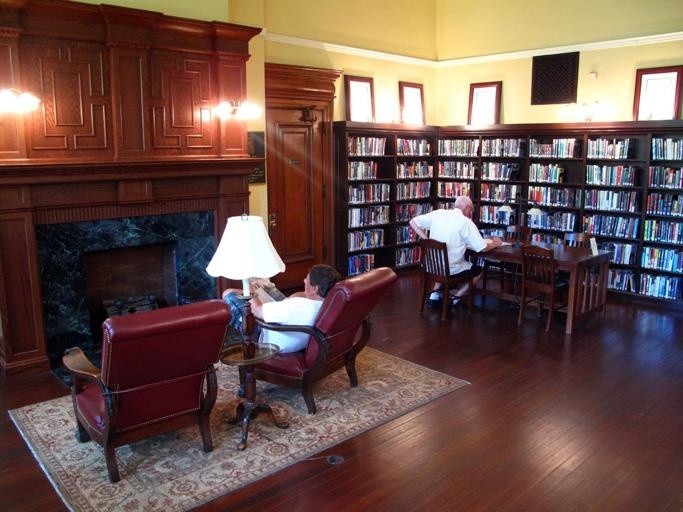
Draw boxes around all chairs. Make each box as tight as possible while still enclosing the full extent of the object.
[420,238,473,320]
[564,233,587,248]
[484,225,530,289]
[62,297,232,483]
[519,246,568,333]
[238,266,397,414]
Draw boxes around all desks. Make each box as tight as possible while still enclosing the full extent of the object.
[467,238,614,335]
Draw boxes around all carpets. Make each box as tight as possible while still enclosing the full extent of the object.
[8,342,470,512]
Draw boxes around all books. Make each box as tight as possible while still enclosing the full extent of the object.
[346,133,683,299]
[249,278,286,305]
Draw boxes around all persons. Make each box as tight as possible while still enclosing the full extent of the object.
[221,263,341,356]
[407,195,502,316]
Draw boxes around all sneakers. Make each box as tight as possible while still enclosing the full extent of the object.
[448,298,468,312]
[427,297,441,310]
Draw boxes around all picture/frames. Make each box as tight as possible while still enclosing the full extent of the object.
[344,76,375,122]
[399,82,425,126]
[632,66,683,121]
[468,80,501,125]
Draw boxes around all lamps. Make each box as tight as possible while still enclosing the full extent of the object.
[498,193,541,226]
[206,214,288,449]
